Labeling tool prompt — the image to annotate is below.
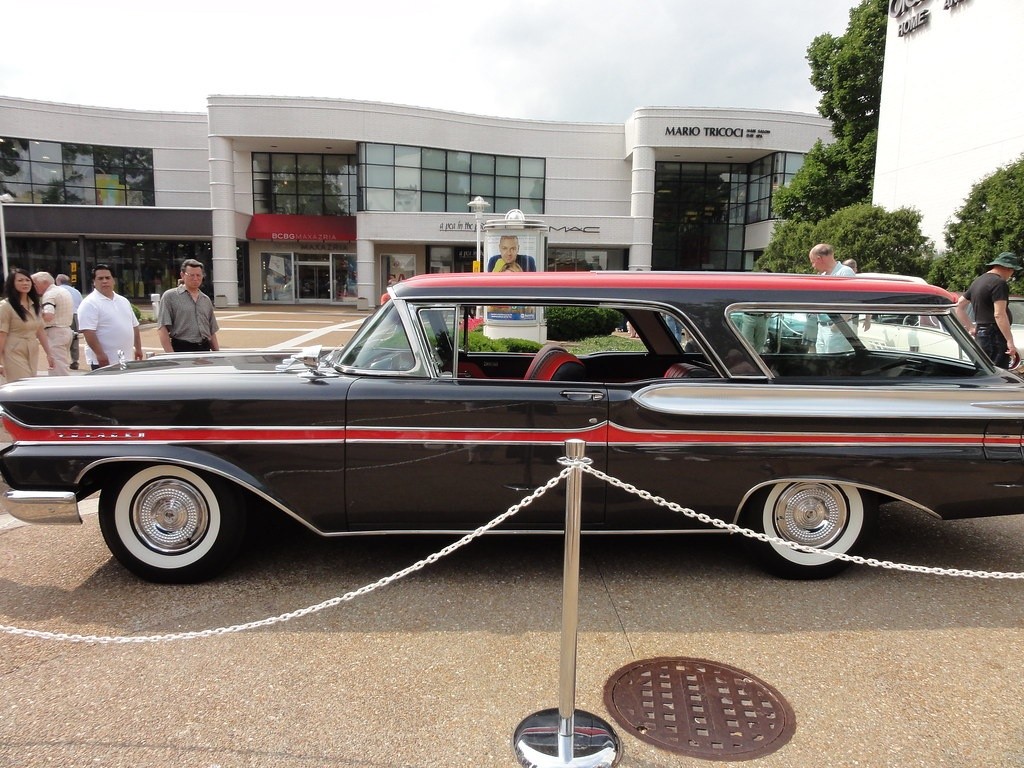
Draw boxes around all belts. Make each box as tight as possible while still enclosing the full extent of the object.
[744,312,764,317]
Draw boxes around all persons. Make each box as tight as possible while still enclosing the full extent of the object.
[809,243,870,354]
[742,312,773,355]
[157,259,220,352]
[75,264,143,370]
[955,253,1023,371]
[0,268,55,384]
[30,271,74,376]
[55,274,83,370]
[619,313,681,342]
[488,236,536,272]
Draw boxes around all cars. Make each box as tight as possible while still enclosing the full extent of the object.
[955,296,1024,373]
[0,273,1024,585]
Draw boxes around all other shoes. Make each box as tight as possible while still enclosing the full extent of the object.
[70,362,78,370]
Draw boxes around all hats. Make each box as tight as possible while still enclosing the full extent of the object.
[985,252,1024,271]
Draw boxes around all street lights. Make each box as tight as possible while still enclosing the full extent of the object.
[467,196,491,273]
[0,193,16,282]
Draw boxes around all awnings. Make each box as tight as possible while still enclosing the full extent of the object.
[246,213,357,242]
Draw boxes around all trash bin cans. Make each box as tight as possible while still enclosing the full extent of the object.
[357,297,369,311]
[215,294,227,309]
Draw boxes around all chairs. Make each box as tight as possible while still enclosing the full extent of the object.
[662,363,714,379]
[523,344,567,379]
[535,353,584,381]
